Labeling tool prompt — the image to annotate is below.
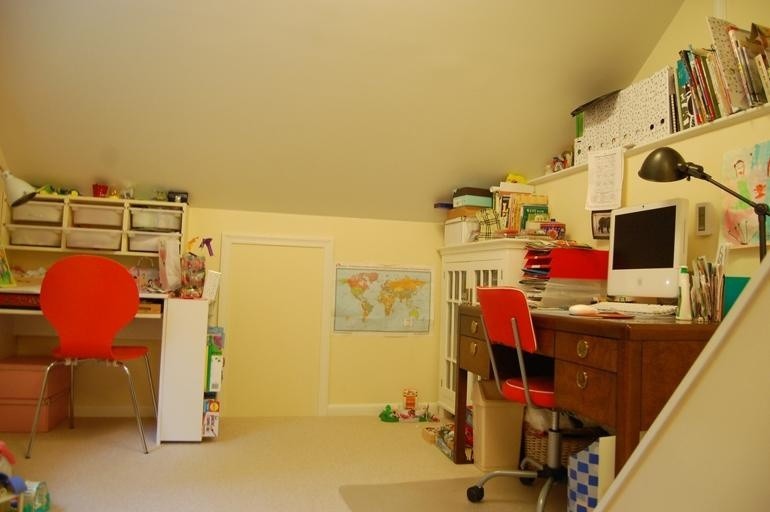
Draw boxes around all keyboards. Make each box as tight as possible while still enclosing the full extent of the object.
[593,301,677,316]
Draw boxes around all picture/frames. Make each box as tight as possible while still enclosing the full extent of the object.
[719,141,770,249]
[335,268,431,332]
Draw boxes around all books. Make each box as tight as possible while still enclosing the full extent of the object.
[496,189,591,248]
[671,17,766,133]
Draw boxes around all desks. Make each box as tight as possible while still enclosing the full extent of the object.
[0,195,186,447]
[454,302,722,479]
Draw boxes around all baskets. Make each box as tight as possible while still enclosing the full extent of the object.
[515,426,593,472]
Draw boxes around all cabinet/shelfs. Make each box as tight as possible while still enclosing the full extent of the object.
[161,298,215,442]
[437,238,533,418]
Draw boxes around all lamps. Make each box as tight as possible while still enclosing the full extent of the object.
[637,147,769,261]
[1,169,39,207]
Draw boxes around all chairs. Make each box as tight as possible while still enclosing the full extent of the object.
[24,256,157,460]
[467,286,558,509]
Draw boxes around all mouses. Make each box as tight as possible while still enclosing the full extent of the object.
[569,303,599,315]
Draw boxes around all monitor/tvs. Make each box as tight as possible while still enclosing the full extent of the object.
[607,197,689,299]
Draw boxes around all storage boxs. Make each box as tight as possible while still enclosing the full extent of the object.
[523,419,598,467]
[69,203,123,227]
[0,398,67,432]
[127,230,181,252]
[65,228,122,249]
[128,207,182,230]
[12,201,64,222]
[0,358,68,398]
[7,226,61,247]
[444,215,480,245]
